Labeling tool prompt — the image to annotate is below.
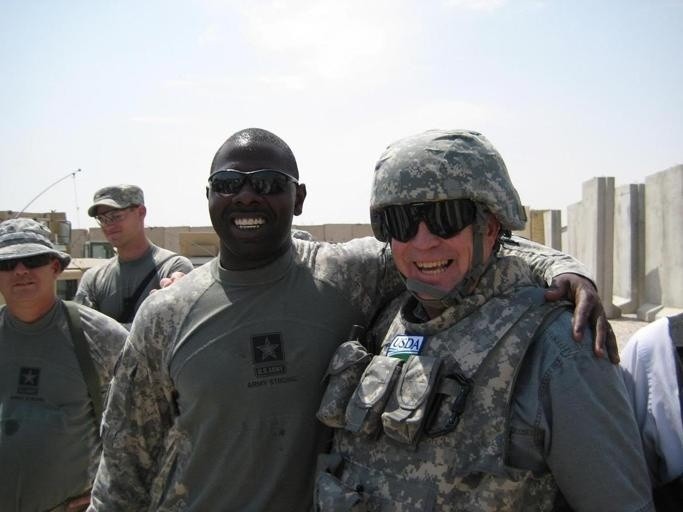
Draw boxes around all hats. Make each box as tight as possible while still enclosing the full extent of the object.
[0,216,72,272]
[87,183,145,216]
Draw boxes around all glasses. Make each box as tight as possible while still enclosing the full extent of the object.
[378,198,490,243]
[95,204,138,227]
[206,167,303,194]
[0,253,53,272]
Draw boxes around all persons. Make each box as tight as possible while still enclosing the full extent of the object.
[71,185,194,333]
[84,128,618,511]
[0,217,130,511]
[622,311,681,511]
[310,128,655,509]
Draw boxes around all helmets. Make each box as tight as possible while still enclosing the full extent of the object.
[368,125,526,233]
